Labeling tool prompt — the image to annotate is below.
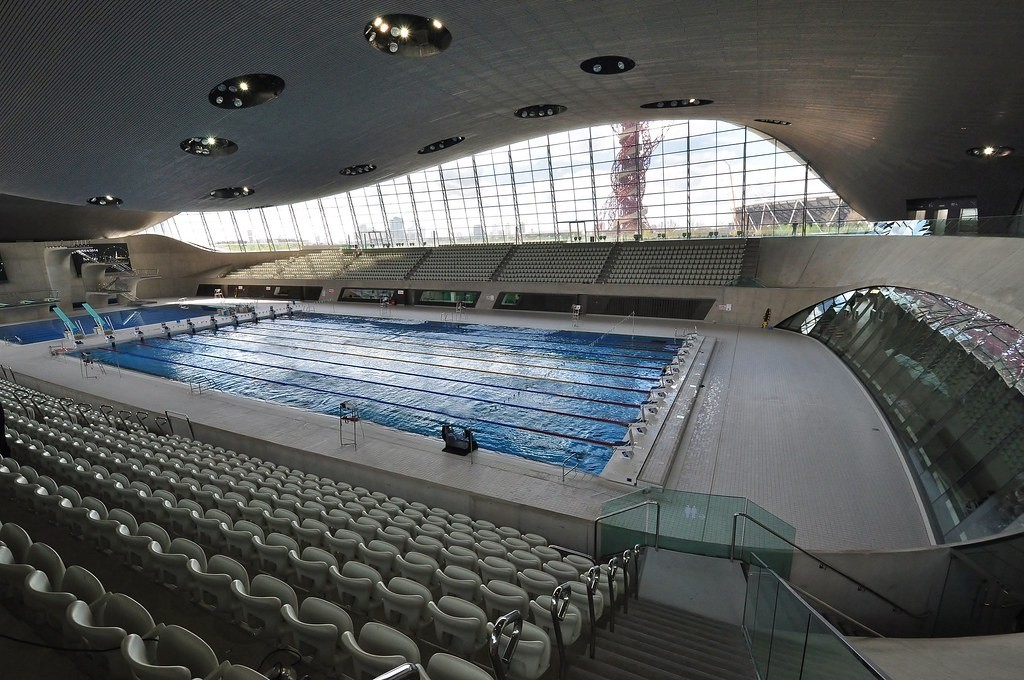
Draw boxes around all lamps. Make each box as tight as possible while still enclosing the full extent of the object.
[85,13,626,206]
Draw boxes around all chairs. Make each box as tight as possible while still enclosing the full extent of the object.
[220,240,748,286]
[0,379,633,679]
[214,289,223,298]
[78,350,107,378]
